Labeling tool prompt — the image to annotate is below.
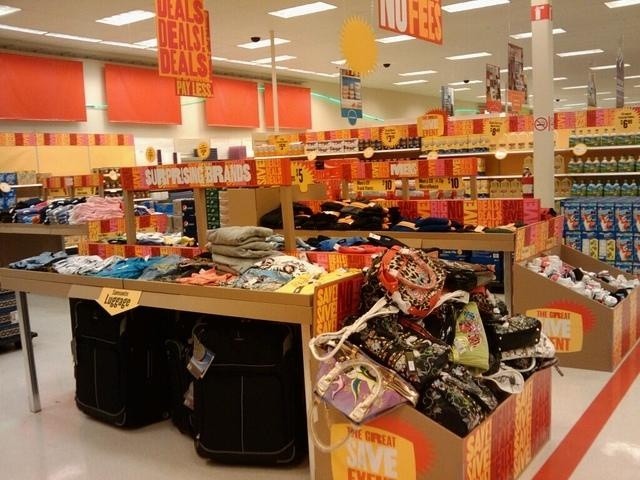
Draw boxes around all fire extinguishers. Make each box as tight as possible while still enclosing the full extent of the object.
[522,167,533,198]
